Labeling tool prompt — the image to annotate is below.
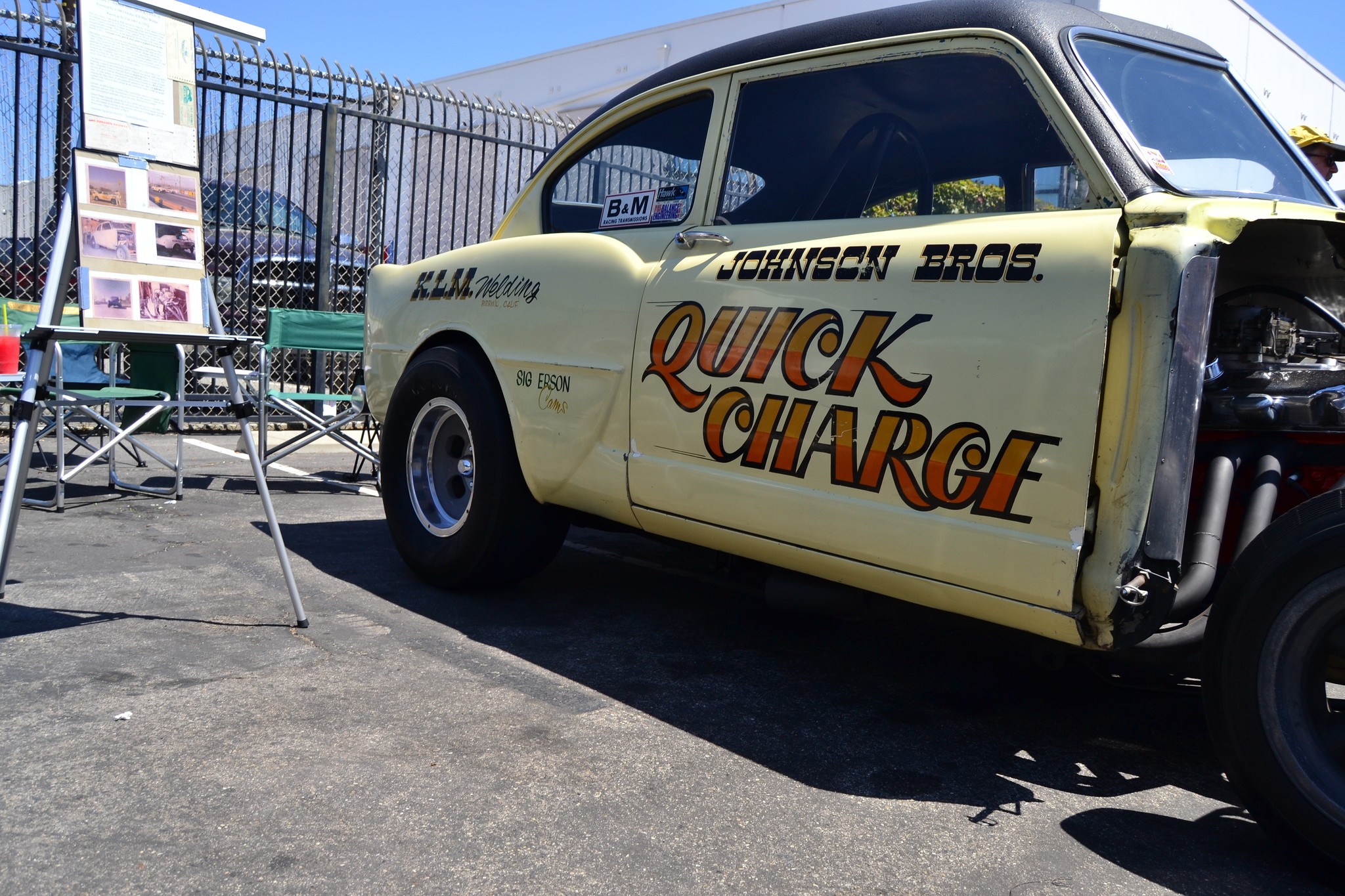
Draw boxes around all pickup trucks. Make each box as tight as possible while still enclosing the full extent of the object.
[0,180,373,388]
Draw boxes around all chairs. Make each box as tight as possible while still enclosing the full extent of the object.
[0,298,185,512]
[259,307,383,497]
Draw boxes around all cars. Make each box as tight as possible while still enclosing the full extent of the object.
[359,0,1345,873]
